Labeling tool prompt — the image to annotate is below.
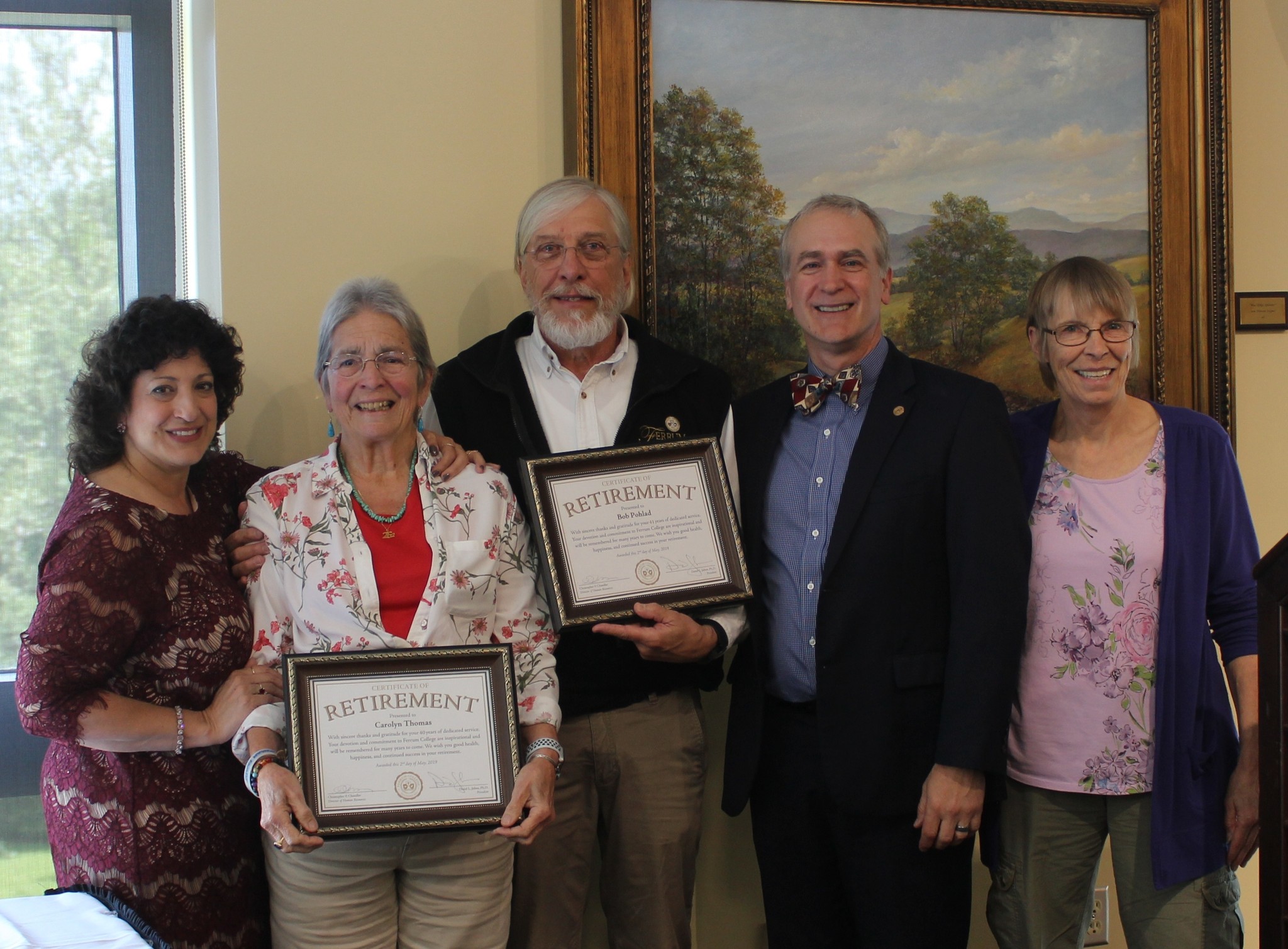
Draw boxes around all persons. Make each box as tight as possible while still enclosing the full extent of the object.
[223,175,752,949]
[721,194,1031,949]
[229,283,566,949]
[981,255,1261,949]
[13,295,502,949]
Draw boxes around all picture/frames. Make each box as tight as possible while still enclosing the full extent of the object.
[563,2,1236,489]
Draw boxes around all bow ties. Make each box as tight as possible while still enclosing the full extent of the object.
[789,364,862,417]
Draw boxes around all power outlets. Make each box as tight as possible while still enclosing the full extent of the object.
[1083,885,1110,947]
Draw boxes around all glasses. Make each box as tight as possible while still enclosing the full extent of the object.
[324,351,417,378]
[523,240,621,268]
[1042,321,1137,346]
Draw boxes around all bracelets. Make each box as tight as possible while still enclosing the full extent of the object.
[243,749,288,798]
[175,706,185,755]
[525,737,564,780]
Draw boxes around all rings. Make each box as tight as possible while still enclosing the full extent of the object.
[250,667,255,674]
[258,683,268,695]
[955,823,970,832]
[272,837,284,849]
